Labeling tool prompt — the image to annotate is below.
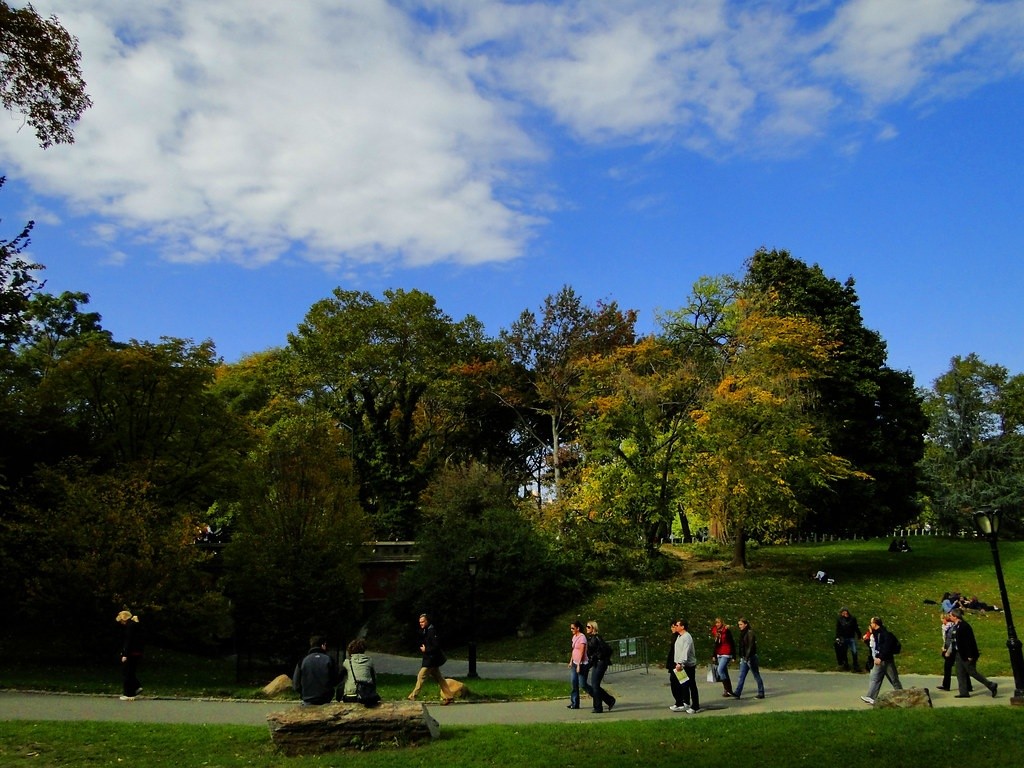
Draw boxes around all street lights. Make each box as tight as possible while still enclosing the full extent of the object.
[969,503,1024,696]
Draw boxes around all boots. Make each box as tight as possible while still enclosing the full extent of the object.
[722,679,733,696]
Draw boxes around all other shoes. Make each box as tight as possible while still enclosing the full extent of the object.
[608,700,615,711]
[955,693,971,697]
[591,710,603,713]
[669,704,684,714]
[939,686,950,691]
[726,692,740,699]
[135,687,143,694]
[567,705,579,708]
[991,683,998,697]
[860,696,874,706]
[685,703,701,713]
[753,694,764,699]
[120,696,138,700]
[441,697,454,705]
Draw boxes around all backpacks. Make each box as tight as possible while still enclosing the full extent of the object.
[886,632,901,654]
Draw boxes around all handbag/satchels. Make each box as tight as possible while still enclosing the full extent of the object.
[438,651,447,667]
[358,681,381,707]
[706,661,722,682]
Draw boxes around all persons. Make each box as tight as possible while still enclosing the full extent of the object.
[710,616,736,697]
[666,619,703,714]
[860,616,902,704]
[407,613,454,705]
[117,608,145,700]
[936,593,1000,698]
[567,620,592,709]
[887,540,901,552]
[291,635,343,705]
[835,606,862,671]
[344,640,376,701]
[727,618,765,699]
[899,537,912,552]
[811,570,835,585]
[586,621,616,713]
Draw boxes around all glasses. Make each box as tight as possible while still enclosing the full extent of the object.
[571,628,578,630]
[586,626,593,629]
